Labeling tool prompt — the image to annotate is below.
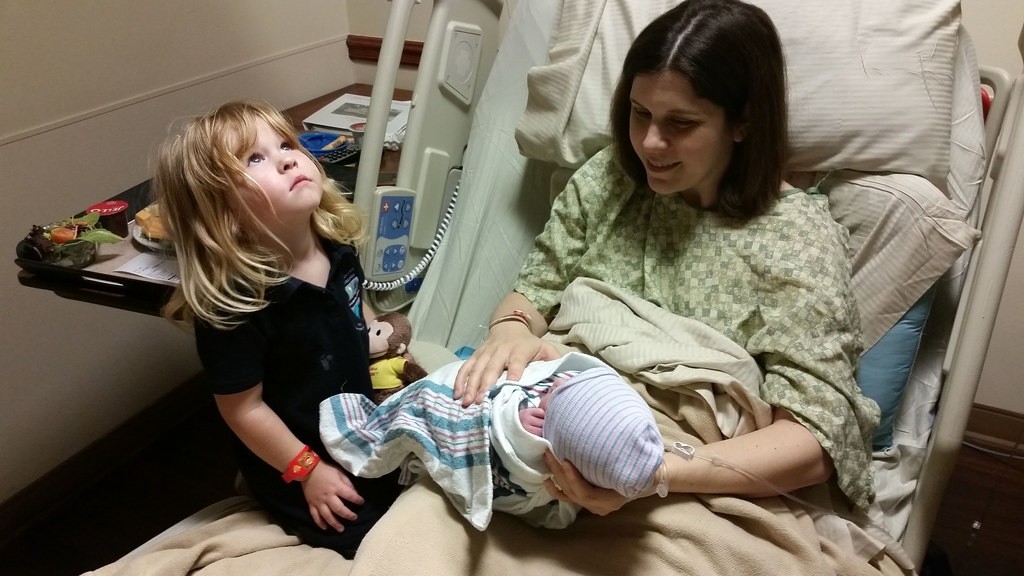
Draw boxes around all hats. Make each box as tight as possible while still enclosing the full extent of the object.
[543,367,668,499]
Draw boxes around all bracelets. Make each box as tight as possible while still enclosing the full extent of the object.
[487,310,535,334]
[282,445,321,485]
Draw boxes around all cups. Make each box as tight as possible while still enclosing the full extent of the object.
[85,199,130,239]
[348,123,367,149]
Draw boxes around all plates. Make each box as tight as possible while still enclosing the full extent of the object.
[132,223,174,253]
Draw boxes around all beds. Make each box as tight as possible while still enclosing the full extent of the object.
[74,0,1024,576]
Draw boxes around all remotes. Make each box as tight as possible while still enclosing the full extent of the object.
[317,141,363,168]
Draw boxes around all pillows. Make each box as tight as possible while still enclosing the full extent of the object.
[515,0,962,195]
[548,161,977,452]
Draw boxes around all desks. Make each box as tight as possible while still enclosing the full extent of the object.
[12,82,416,323]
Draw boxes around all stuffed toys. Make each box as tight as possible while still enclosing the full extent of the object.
[366,312,428,407]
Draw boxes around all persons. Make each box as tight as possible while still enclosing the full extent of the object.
[159,100,417,559]
[319,0,879,532]
